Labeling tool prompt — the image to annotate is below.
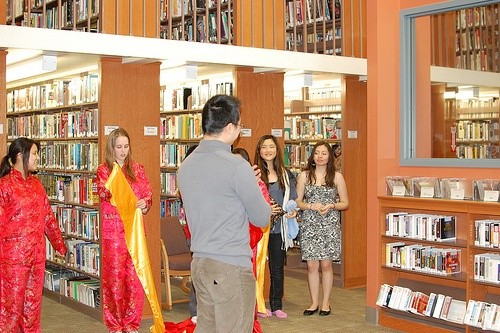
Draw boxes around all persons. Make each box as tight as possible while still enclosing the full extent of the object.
[0,97,355,333]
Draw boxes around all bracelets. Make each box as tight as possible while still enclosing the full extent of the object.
[332,204,335,209]
[310,203,313,210]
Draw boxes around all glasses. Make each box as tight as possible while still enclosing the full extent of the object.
[224,121,244,130]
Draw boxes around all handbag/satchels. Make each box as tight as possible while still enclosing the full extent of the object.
[285,200,299,239]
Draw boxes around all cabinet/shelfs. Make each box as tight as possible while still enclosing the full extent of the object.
[0,0,500,333]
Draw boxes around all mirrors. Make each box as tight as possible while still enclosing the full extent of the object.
[400,0,500,168]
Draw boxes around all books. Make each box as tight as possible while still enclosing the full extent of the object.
[7,0,500,332]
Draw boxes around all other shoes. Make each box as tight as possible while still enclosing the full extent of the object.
[319,305,331,316]
[303,307,318,316]
[191,316,197,323]
[273,309,288,319]
[257,308,272,318]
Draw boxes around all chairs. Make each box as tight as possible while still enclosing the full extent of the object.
[160,216,193,312]
